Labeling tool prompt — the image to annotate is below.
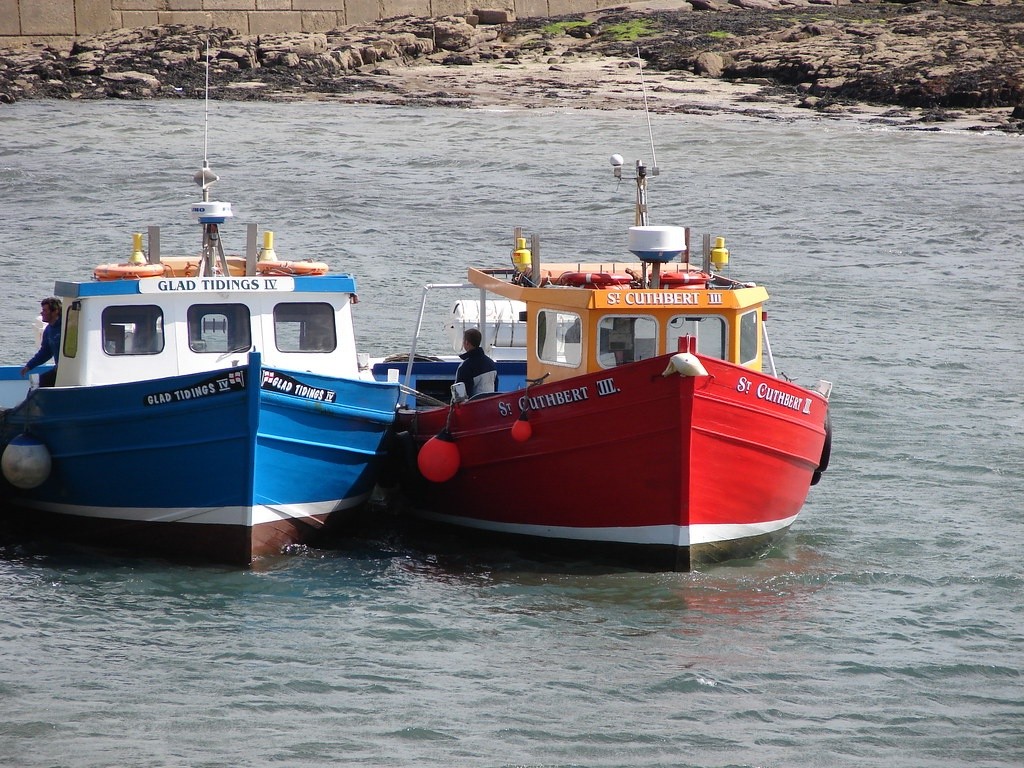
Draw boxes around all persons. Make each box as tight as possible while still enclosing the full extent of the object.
[456,329,499,399]
[21,297,62,387]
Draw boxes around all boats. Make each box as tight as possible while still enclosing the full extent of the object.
[0,33,403,570]
[396,47,831,571]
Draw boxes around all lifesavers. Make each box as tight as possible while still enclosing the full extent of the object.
[562,274,631,292]
[96,263,166,278]
[648,270,710,291]
[256,258,326,277]
